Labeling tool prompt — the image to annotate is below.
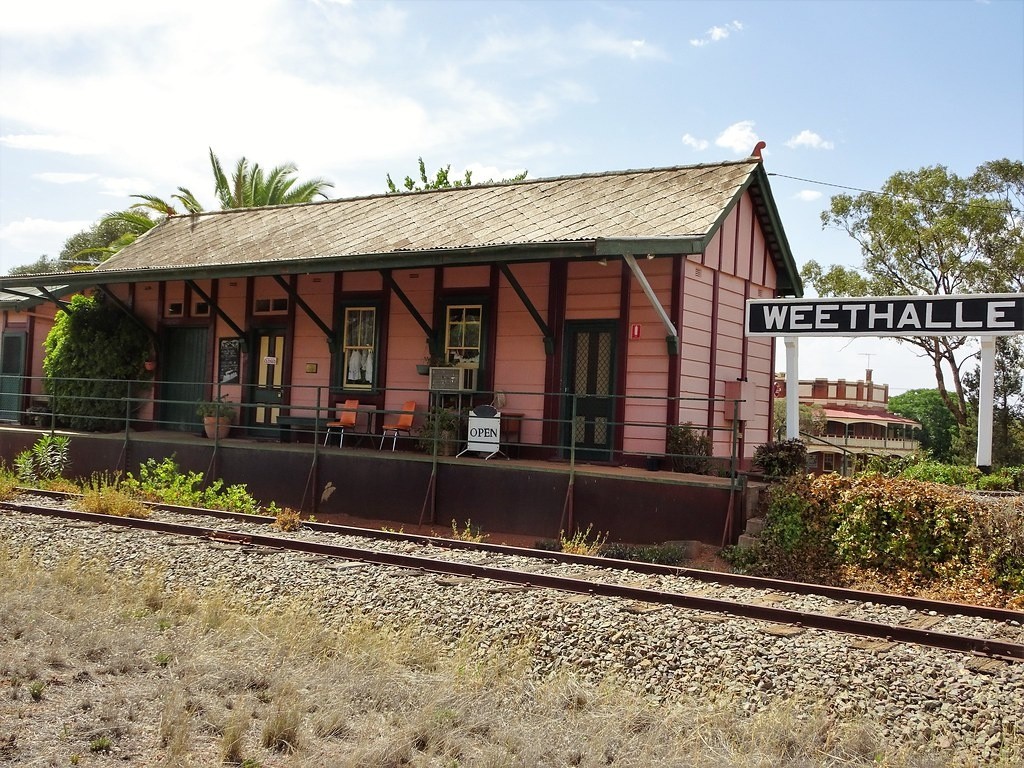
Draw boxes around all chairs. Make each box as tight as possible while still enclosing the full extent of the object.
[380,401,416,452]
[324,400,359,448]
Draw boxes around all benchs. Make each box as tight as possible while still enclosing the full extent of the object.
[275,415,341,446]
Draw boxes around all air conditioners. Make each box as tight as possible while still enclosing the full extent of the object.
[429,367,477,391]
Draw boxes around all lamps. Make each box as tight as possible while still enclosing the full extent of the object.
[599,257,607,266]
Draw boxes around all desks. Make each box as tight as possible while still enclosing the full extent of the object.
[500,412,526,459]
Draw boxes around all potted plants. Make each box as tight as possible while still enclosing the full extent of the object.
[196,393,236,439]
[425,337,444,367]
[142,349,156,370]
[417,408,470,456]
[415,357,431,376]
